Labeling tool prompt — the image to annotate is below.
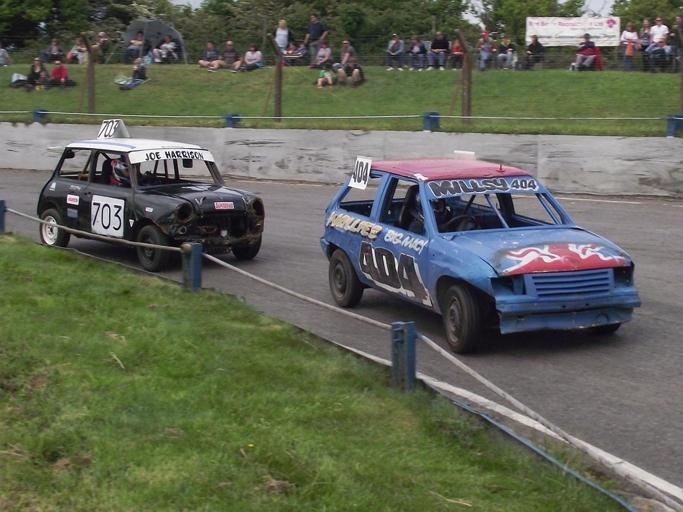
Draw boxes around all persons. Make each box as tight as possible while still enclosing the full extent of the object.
[409,192,478,234]
[198,14,365,89]
[574,33,597,72]
[106,158,143,187]
[386,31,545,72]
[25,30,179,92]
[621,15,683,73]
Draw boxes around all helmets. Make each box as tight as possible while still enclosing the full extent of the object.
[415,193,446,224]
[111,159,142,185]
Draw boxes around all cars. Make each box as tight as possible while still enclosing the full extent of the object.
[319,156,640,354]
[36,118,265,270]
